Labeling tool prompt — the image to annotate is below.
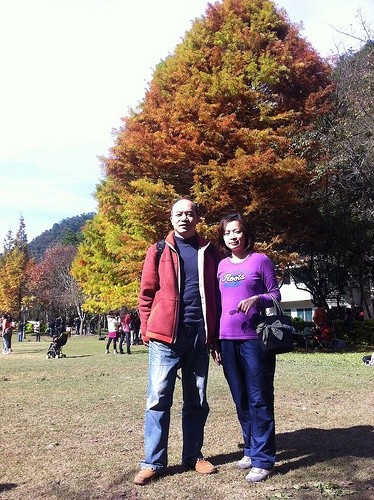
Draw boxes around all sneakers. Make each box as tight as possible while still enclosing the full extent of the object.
[236,456,252,469]
[246,467,272,483]
[134,468,158,484]
[189,456,216,474]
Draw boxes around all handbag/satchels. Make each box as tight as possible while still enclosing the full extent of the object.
[5,328,12,334]
[259,295,295,354]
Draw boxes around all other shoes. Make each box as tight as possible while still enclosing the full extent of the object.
[105,350,109,354]
[127,352,131,354]
[120,352,125,354]
[114,350,118,354]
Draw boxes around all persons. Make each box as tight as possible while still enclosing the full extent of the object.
[102,309,142,354]
[0,312,27,354]
[46,315,83,359]
[208,212,283,482]
[33,319,41,342]
[133,197,225,485]
[314,303,335,343]
